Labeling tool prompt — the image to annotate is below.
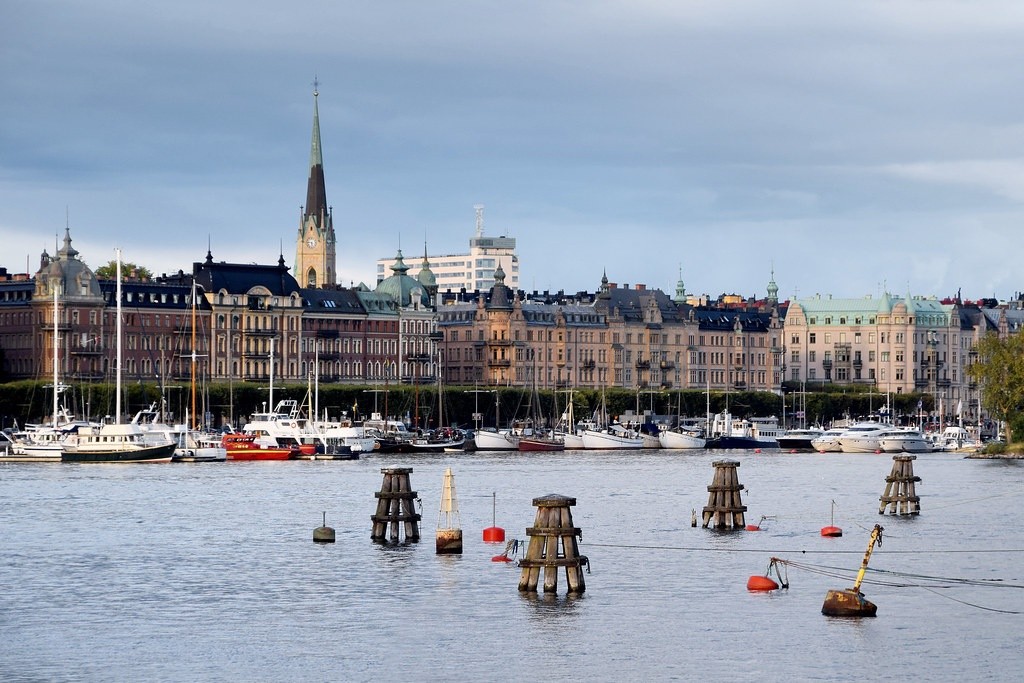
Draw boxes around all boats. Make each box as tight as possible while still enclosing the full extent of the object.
[0,245,1010,476]
